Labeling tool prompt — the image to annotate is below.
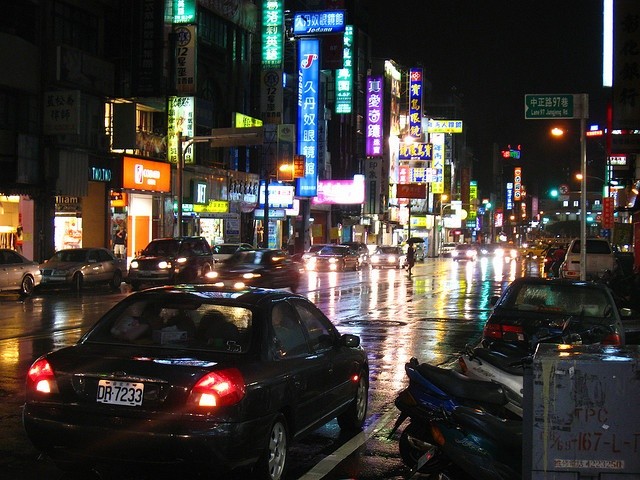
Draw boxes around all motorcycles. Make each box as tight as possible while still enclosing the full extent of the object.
[464,305,617,372]
[387,358,523,477]
[481,315,583,353]
[458,352,525,419]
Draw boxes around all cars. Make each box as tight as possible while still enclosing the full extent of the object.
[309,244,359,272]
[500,243,520,259]
[341,242,369,268]
[211,243,257,270]
[39,247,127,293]
[0,249,42,298]
[22,281,369,480]
[402,245,426,261]
[480,275,630,348]
[452,244,477,262]
[370,245,407,269]
[542,247,557,276]
[479,244,500,258]
[204,249,300,292]
[438,241,459,256]
[559,237,617,280]
[521,242,544,258]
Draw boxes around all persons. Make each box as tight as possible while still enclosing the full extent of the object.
[406,243,416,275]
[287,234,297,254]
[113,223,127,258]
[13,225,23,254]
[554,246,565,259]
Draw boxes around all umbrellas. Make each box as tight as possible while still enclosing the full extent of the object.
[406,237,425,243]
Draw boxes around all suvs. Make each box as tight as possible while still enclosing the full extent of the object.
[125,236,211,289]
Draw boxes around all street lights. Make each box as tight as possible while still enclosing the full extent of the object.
[432,195,449,256]
[574,173,614,198]
[261,164,291,248]
[549,127,613,199]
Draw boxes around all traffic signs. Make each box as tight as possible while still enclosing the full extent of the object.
[525,95,574,121]
